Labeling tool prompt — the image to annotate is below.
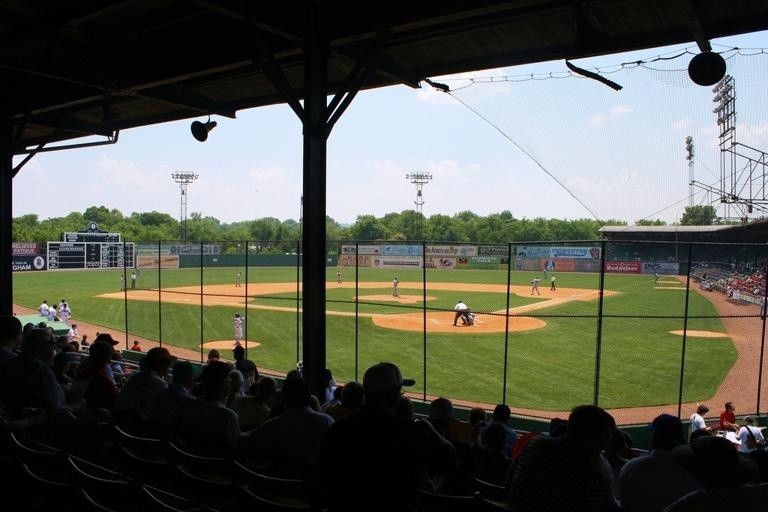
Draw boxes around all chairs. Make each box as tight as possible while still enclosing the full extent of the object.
[689,263,768,306]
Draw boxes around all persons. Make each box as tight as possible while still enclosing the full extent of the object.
[336,270,342,284]
[131,273,136,288]
[120,274,124,291]
[531,278,540,295]
[453,300,474,325]
[550,273,556,290]
[2,316,767,510]
[393,277,398,296]
[236,271,241,287]
[701,255,767,316]
[39,298,80,341]
[234,313,243,344]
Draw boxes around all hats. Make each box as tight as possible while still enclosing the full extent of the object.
[362,362,416,395]
[146,347,178,364]
[97,335,120,346]
